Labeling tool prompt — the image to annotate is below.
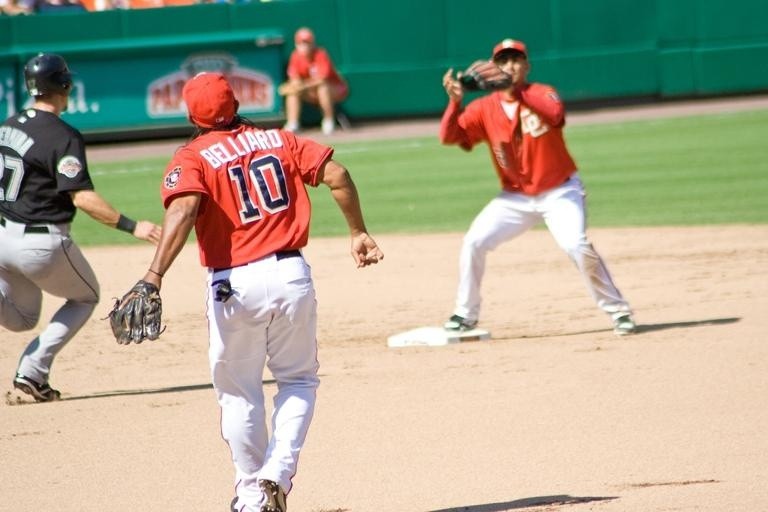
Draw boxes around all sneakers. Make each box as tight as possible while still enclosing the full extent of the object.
[441,315,478,338]
[13,373,59,402]
[613,316,638,336]
[231,478,286,511]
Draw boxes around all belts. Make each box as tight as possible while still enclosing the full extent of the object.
[216,250,301,273]
[0,218,48,233]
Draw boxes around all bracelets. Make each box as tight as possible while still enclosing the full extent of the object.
[114,215,136,233]
[148,268,163,277]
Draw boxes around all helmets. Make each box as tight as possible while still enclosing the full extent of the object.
[25,54,78,97]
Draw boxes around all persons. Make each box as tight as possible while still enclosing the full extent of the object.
[277,27,348,136]
[111,71,384,512]
[438,38,635,341]
[1,54,162,403]
[1,1,95,20]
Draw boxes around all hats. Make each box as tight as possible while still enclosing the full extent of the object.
[493,39,527,59]
[296,29,314,45]
[182,72,235,129]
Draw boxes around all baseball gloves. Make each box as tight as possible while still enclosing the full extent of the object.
[460,60,511,90]
